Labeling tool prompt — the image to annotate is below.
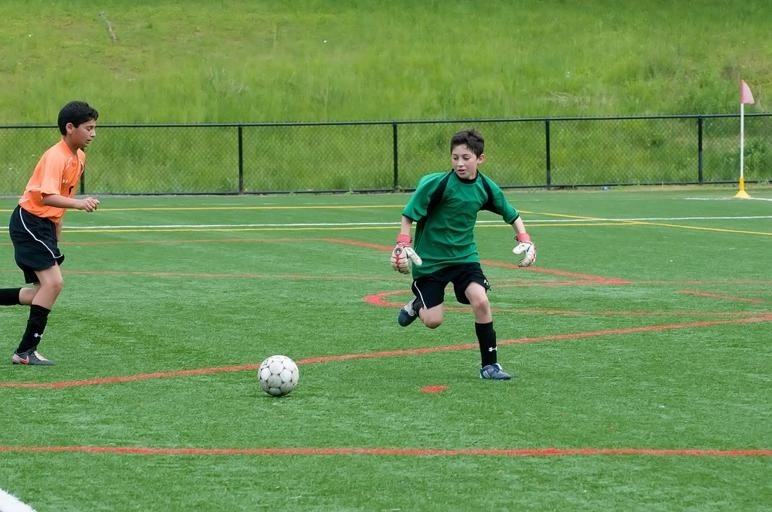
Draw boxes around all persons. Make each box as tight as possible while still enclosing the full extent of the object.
[390,129,537,380]
[0,100,100,365]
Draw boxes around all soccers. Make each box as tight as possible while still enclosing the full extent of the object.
[258,355,300,396]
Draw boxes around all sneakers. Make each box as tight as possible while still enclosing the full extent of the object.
[398,295,417,326]
[479,363,511,379]
[12,348,56,366]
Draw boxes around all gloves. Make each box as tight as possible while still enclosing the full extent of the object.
[512,233,537,268]
[390,234,423,275]
